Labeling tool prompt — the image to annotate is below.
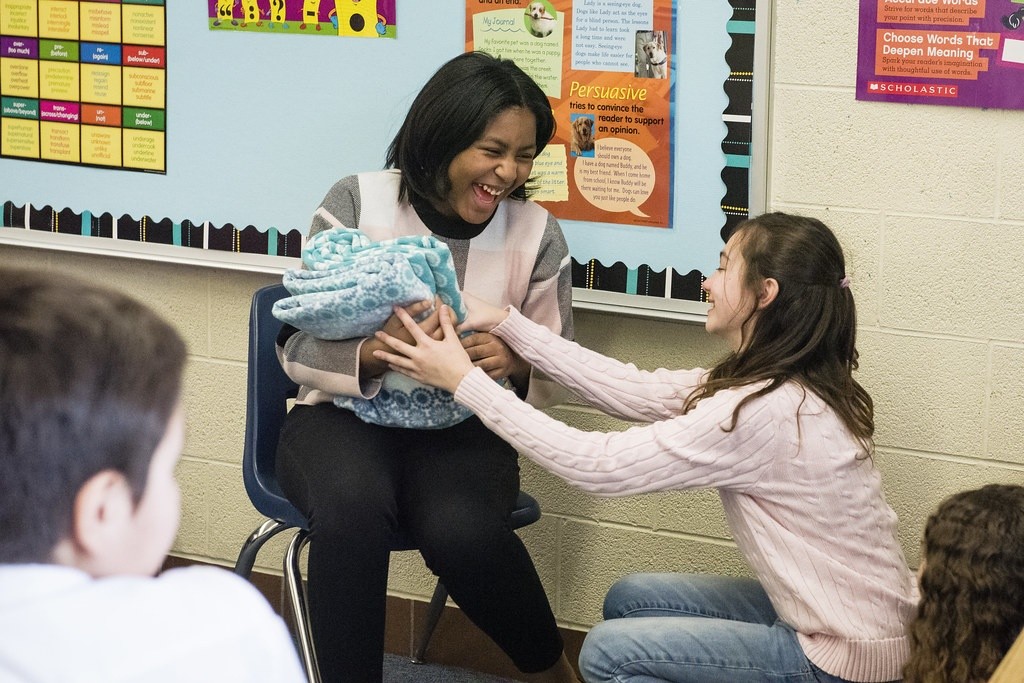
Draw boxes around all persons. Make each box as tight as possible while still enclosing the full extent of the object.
[370,211,916,683]
[0,269,305,683]
[272,55,583,682]
[901,484,1024,683]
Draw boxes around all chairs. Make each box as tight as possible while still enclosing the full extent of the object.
[234,284,541,683]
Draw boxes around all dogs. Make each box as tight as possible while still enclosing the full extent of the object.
[529,3,556,38]
[644,42,667,79]
[571,116,594,156]
